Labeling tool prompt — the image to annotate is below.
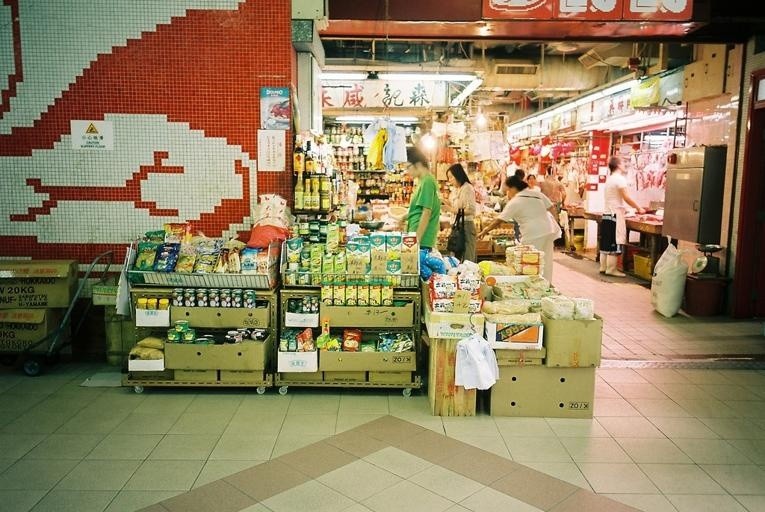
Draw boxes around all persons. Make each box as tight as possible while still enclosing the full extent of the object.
[475,172,560,286]
[441,163,478,264]
[598,156,647,277]
[512,168,528,242]
[390,146,442,254]
[536,166,567,223]
[525,174,541,192]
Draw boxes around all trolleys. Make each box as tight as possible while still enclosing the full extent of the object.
[0,251,114,376]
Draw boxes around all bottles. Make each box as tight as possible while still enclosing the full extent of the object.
[291,135,346,245]
[384,163,413,206]
[137,298,170,310]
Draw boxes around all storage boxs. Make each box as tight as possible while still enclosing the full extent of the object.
[681,273,727,316]
[662,145,726,245]
[0,260,78,309]
[484,319,546,366]
[542,308,603,368]
[490,365,595,420]
[0,310,74,358]
[420,261,542,337]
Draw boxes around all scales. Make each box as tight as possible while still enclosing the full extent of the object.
[692,244,723,277]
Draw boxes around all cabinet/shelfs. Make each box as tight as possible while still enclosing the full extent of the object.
[274,232,422,397]
[126,230,274,395]
[517,117,674,280]
[322,116,516,261]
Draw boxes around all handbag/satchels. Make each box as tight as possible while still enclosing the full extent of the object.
[446,208,467,252]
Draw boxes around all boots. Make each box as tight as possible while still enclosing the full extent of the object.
[599,252,606,272]
[605,255,626,278]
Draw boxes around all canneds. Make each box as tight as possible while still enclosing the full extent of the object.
[280,330,302,352]
[298,218,328,243]
[171,288,256,308]
[166,320,216,345]
[309,172,332,211]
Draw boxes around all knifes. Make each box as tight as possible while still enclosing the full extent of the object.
[635,210,657,215]
